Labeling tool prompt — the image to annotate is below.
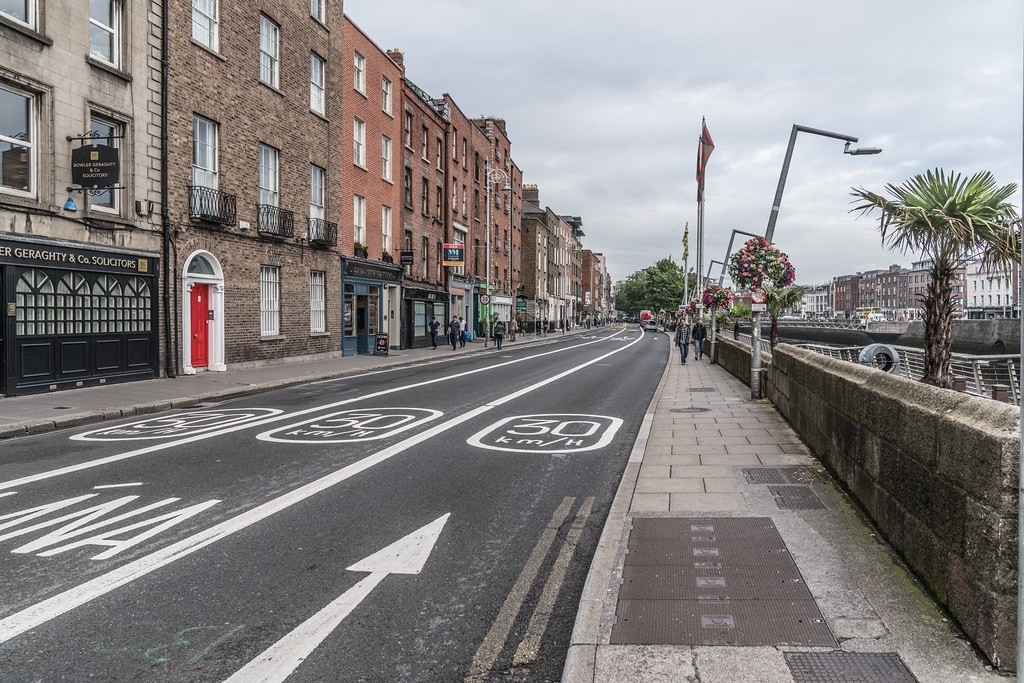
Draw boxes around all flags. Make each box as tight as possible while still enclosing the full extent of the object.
[681,223,689,259]
[696,116,715,202]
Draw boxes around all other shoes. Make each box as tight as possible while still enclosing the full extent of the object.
[695,357,697,360]
[699,357,702,360]
[433,346,436,350]
[500,346,501,349]
[460,346,462,348]
[681,361,685,364]
[498,347,499,350]
[463,344,465,347]
[453,348,456,350]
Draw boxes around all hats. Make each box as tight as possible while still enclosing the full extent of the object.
[697,317,701,320]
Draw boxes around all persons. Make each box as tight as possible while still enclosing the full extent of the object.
[692,318,707,361]
[536,318,541,336]
[446,316,467,351]
[542,317,548,337]
[494,321,505,350]
[427,317,441,350]
[674,317,692,365]
[509,318,518,342]
[581,318,628,328]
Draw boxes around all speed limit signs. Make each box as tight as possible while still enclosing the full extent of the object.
[481,295,490,305]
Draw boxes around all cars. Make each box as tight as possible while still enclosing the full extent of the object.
[644,319,658,332]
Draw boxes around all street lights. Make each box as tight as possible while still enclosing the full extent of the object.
[483,157,512,348]
[709,227,775,366]
[747,122,883,404]
